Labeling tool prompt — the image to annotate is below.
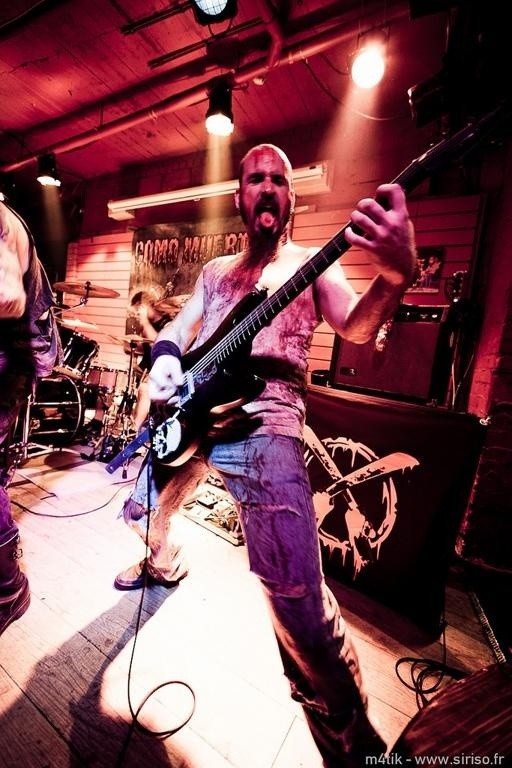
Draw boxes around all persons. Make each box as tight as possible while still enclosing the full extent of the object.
[113,141,418,765]
[419,256,441,288]
[129,291,183,387]
[1,200,64,631]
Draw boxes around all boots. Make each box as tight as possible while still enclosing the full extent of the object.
[0,527,31,637]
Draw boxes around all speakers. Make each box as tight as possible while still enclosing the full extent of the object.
[328,305,451,406]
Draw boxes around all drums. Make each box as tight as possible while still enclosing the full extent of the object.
[82,366,133,397]
[53,325,99,380]
[11,375,82,448]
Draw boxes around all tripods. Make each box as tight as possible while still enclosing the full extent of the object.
[10,293,88,467]
[89,344,149,479]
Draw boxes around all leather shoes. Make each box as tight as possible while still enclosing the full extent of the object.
[115,560,189,591]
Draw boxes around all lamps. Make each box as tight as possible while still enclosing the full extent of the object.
[36,152,61,187]
[205,78,234,137]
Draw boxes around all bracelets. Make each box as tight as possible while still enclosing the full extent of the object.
[147,339,184,365]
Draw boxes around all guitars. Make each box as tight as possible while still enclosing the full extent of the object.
[139,122,475,467]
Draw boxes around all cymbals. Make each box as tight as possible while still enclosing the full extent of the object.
[58,318,98,330]
[116,335,154,342]
[52,281,120,298]
